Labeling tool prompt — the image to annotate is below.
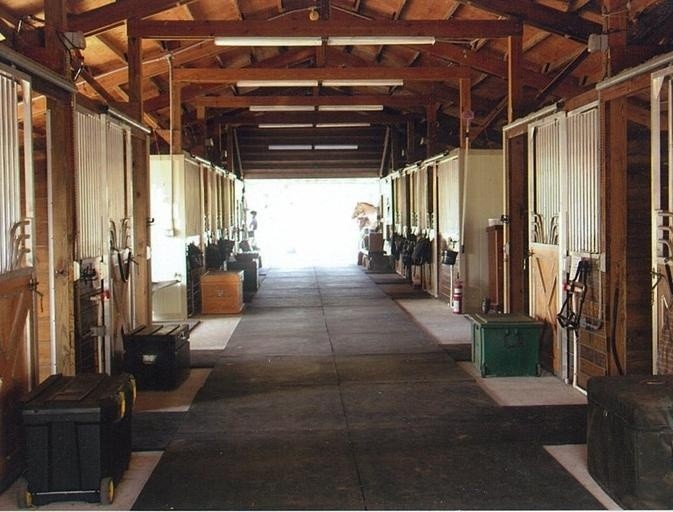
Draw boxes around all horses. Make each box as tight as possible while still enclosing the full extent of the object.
[351,202,379,218]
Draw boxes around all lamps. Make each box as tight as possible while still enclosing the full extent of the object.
[268,145,359,152]
[235,80,403,89]
[250,105,384,112]
[257,123,372,129]
[214,37,436,47]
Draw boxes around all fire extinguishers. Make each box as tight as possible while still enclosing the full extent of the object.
[451,272,462,313]
